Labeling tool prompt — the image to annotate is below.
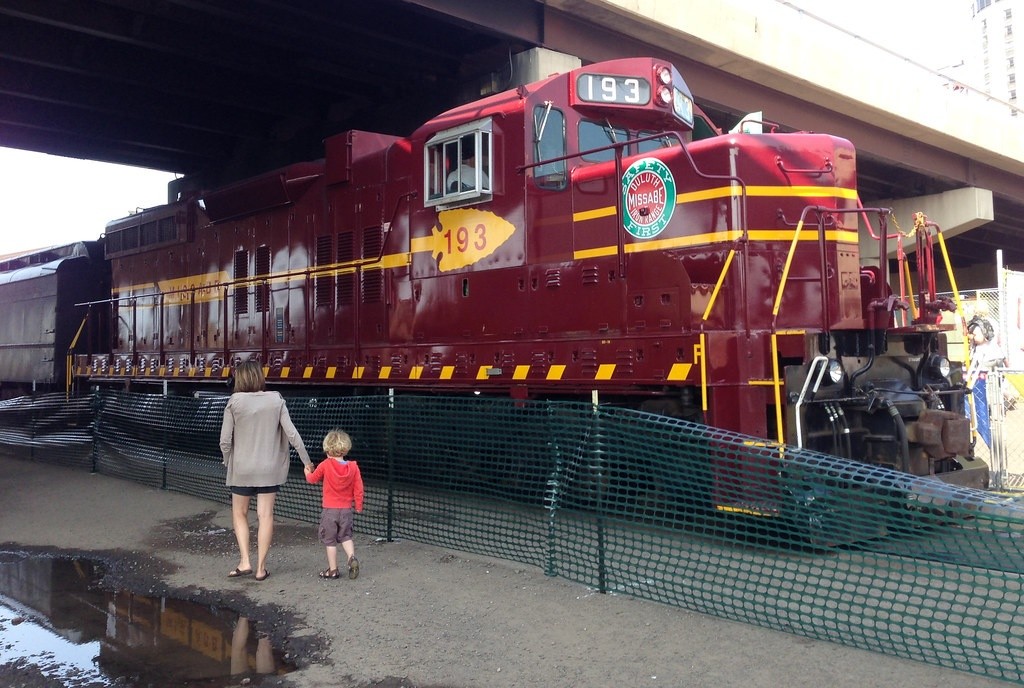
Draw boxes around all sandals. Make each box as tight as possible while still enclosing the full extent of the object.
[319,566,340,579]
[347,556,359,579]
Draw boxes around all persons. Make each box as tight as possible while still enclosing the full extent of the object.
[220,362,314,582]
[304,429,364,578]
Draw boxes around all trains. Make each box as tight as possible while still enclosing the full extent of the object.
[2,56,991,555]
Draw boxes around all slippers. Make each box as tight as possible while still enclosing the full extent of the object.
[228,568,252,577]
[255,568,271,581]
